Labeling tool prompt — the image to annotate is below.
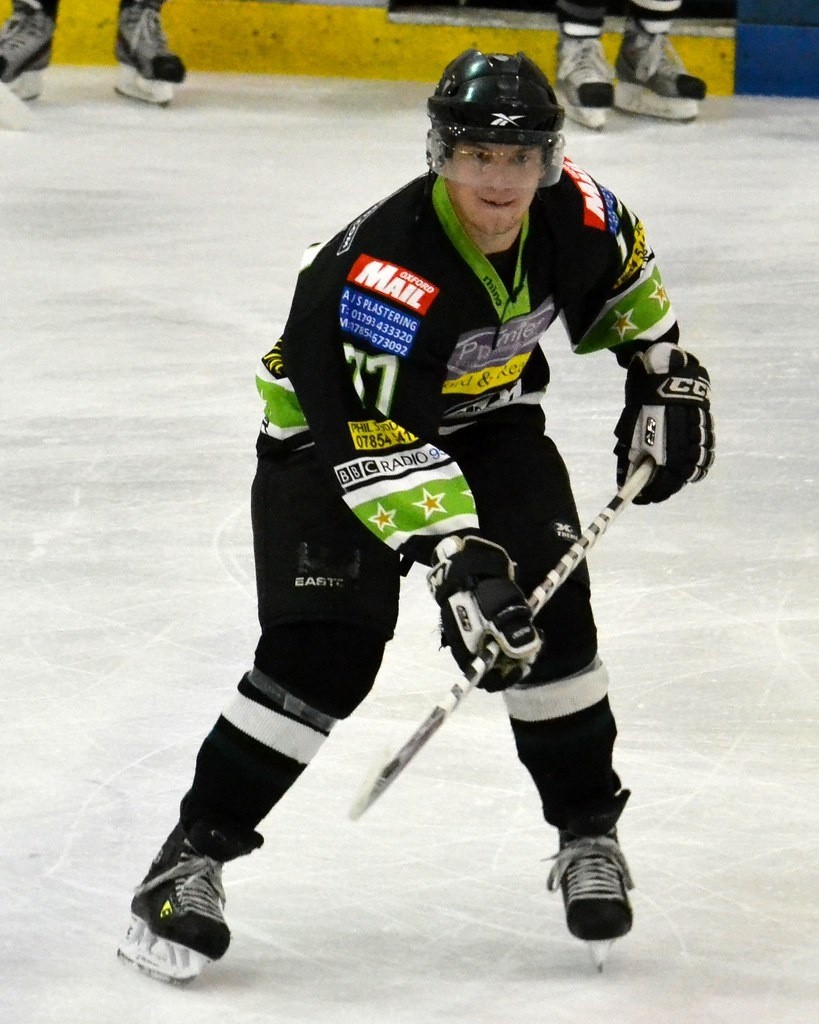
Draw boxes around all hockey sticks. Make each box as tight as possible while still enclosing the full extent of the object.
[340,458,656,824]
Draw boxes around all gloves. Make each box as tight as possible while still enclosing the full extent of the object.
[427,535,544,693]
[613,342,716,505]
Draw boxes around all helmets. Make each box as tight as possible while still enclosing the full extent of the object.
[428,49,566,164]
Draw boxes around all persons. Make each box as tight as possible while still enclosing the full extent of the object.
[0,0,185,104]
[115,49,717,985]
[552,0,707,129]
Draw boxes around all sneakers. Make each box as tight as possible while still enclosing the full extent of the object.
[112,0,186,108]
[117,819,264,987]
[542,790,636,973]
[0,0,59,102]
[553,0,615,130]
[613,0,707,122]
